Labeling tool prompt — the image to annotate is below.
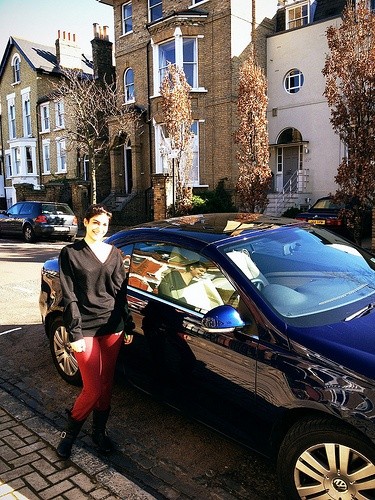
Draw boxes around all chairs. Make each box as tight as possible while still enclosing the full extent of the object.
[224,251,270,292]
[162,247,224,312]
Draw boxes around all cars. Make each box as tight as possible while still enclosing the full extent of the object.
[37,213,375,500]
[0,201,78,243]
[295,194,371,247]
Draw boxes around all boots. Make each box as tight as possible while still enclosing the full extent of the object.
[92,407,117,450]
[57,413,84,458]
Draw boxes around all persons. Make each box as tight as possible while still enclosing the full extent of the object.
[156,259,208,308]
[52,204,135,462]
